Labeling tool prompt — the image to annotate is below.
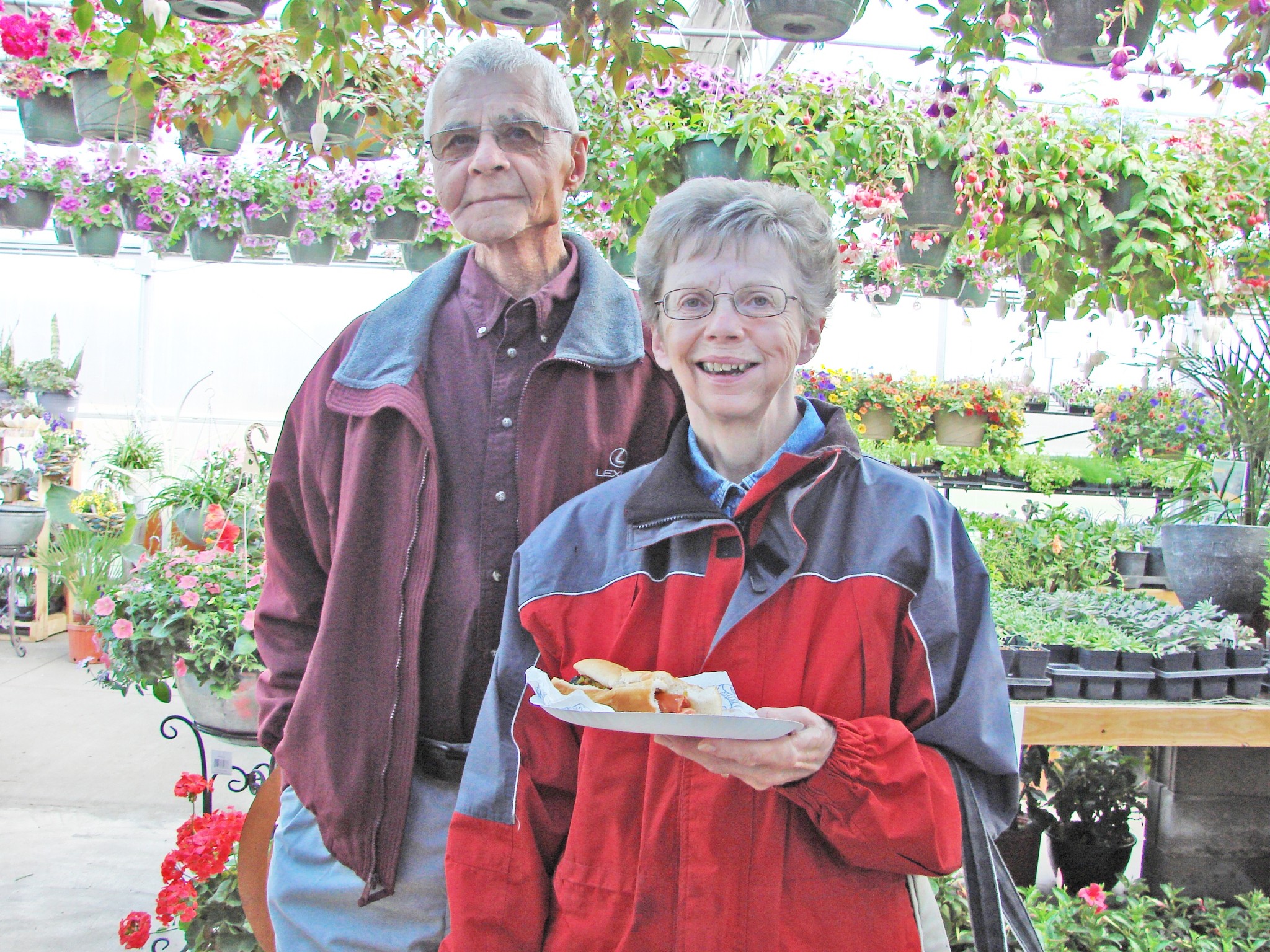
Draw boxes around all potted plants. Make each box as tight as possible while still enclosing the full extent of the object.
[935,328,1269,892]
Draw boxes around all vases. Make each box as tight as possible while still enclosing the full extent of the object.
[891,224,953,272]
[1079,173,1158,274]
[1,505,47,556]
[862,274,903,306]
[174,655,260,747]
[279,74,369,147]
[66,66,166,143]
[15,88,85,147]
[953,278,991,307]
[894,157,969,228]
[172,506,250,546]
[852,401,898,441]
[36,390,81,426]
[0,186,56,231]
[676,136,780,183]
[934,408,988,447]
[916,265,964,299]
[66,624,107,664]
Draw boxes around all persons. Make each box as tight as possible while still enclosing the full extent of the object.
[437,176,1021,952]
[250,36,688,952]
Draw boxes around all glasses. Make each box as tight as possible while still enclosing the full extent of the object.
[653,285,803,320]
[425,121,574,162]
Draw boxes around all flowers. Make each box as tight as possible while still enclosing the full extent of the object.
[0,0,1269,698]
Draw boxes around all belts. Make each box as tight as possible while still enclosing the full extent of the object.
[414,741,467,783]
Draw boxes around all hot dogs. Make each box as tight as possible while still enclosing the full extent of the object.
[551,658,722,715]
